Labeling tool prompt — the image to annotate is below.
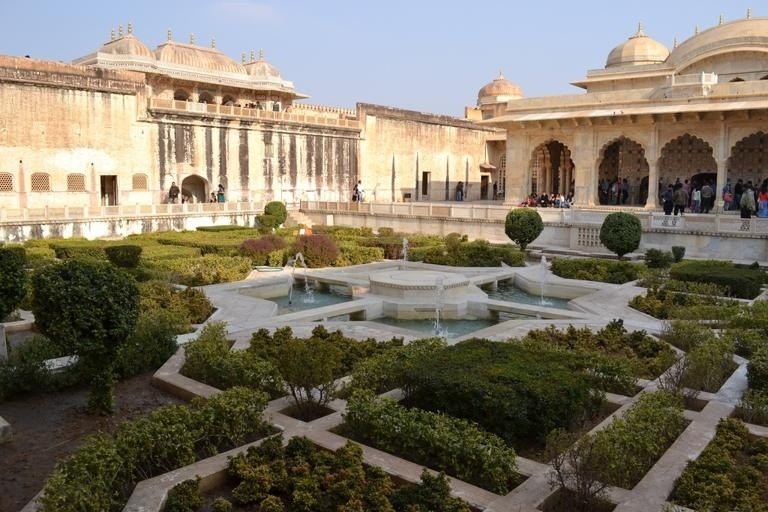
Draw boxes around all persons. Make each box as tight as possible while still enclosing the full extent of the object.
[356,180,365,201]
[660,176,768,219]
[492,180,498,199]
[209,193,216,203]
[169,182,180,204]
[351,184,360,202]
[216,184,225,203]
[456,181,462,201]
[598,177,629,205]
[460,182,464,201]
[519,192,573,208]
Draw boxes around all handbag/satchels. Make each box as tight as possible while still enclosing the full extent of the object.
[353,196,356,201]
